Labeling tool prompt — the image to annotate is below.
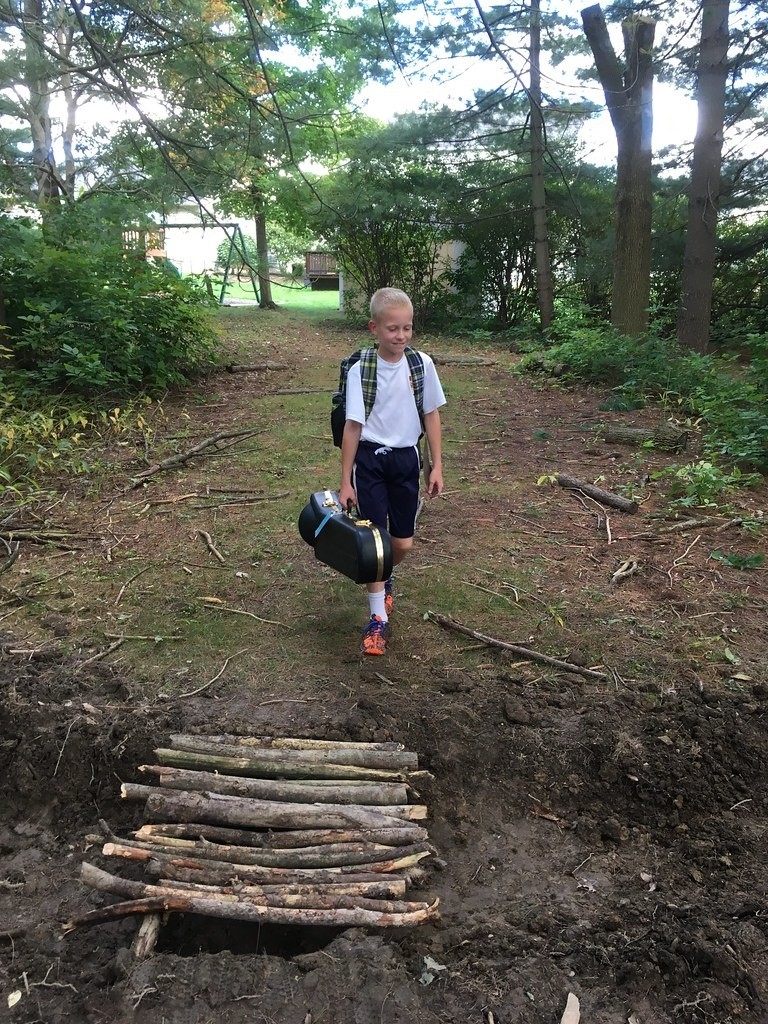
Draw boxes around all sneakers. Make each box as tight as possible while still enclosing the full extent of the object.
[359,614,390,655]
[384,579,394,615]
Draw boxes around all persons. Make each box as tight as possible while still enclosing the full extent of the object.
[339,288,446,654]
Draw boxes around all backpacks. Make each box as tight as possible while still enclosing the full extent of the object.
[330,342,427,451]
